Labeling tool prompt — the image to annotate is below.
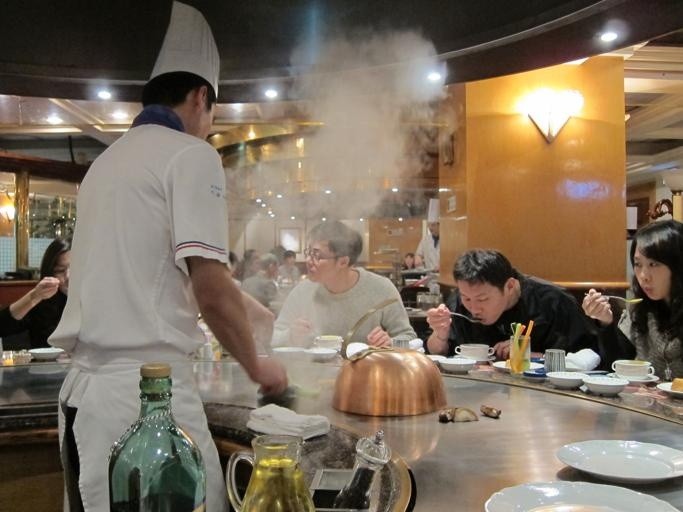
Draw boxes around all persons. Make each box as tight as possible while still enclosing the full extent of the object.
[581,220,682,380]
[424,248,583,361]
[272,221,417,352]
[47,0,289,511]
[228,244,302,314]
[0,236,73,348]
[399,196,442,284]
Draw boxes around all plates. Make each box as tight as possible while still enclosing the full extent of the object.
[567,368,608,375]
[607,371,659,383]
[469,355,494,364]
[655,382,682,398]
[492,360,544,372]
[555,437,683,482]
[482,478,680,511]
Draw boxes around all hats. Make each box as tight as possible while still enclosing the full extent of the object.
[147,1,220,101]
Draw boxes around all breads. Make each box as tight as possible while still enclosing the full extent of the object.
[670,377,683,391]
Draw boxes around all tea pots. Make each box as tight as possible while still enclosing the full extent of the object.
[224,433,319,512]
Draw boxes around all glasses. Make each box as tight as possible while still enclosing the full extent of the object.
[304,249,338,260]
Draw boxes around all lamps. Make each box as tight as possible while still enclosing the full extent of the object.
[526,87,574,145]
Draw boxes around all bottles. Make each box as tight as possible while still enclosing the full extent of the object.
[1,349,29,365]
[333,429,392,506]
[106,362,207,512]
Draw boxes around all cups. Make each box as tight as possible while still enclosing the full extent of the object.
[312,335,343,351]
[453,343,495,357]
[610,358,655,378]
[388,336,411,349]
[508,334,531,379]
[415,291,439,311]
[543,348,565,371]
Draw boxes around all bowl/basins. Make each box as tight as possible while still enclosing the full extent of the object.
[580,376,628,396]
[18,267,39,279]
[438,358,476,374]
[424,353,446,364]
[27,347,62,361]
[273,346,337,361]
[546,371,588,388]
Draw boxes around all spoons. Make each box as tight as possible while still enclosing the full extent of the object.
[582,291,644,306]
[440,308,481,324]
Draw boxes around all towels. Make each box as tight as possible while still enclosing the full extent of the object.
[409,338,425,353]
[247,403,330,440]
[564,348,601,372]
